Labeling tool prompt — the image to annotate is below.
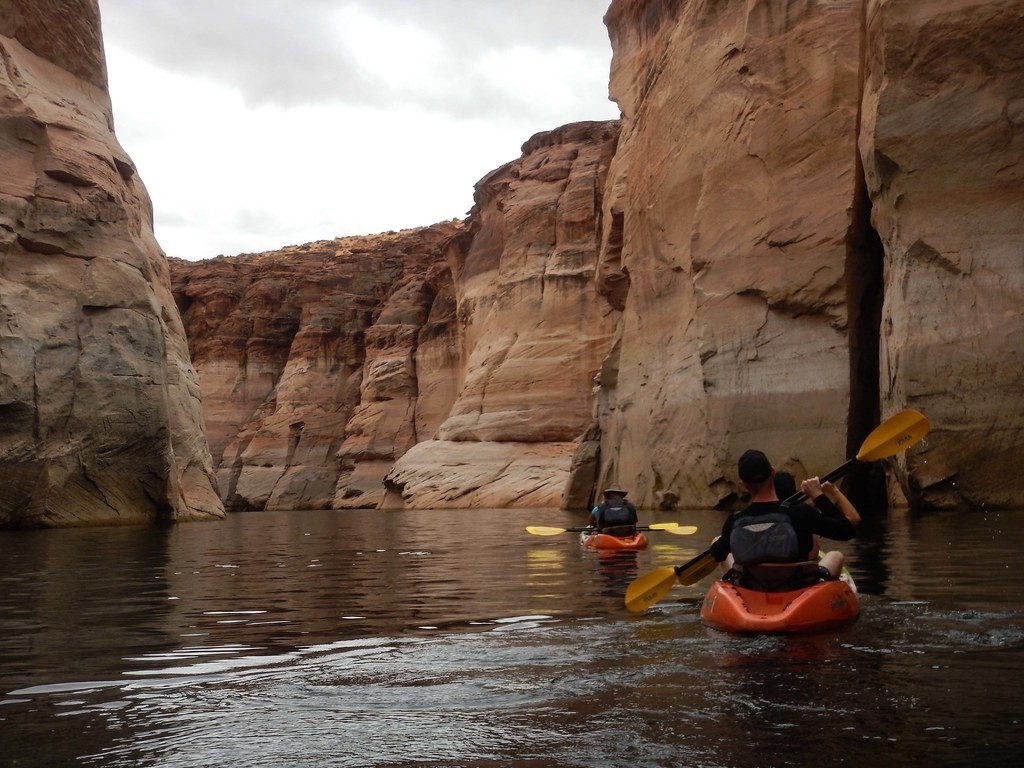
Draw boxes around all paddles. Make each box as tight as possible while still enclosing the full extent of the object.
[624,404,931,613]
[525,522,700,536]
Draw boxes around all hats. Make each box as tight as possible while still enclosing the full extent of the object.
[603,482,627,499]
[738,450,771,483]
[774,472,798,500]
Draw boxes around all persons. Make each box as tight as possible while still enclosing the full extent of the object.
[588,484,638,537]
[711,450,860,591]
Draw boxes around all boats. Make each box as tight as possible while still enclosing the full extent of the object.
[576,524,652,555]
[700,551,862,634]
